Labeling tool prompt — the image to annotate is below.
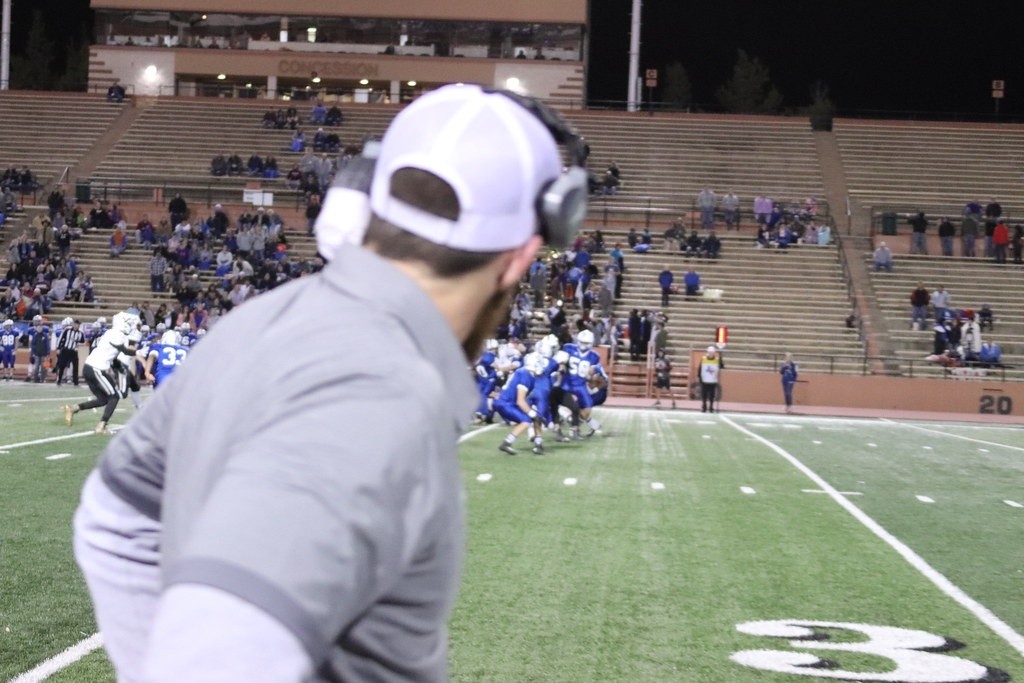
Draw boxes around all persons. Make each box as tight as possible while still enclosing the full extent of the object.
[653,349,676,408]
[260,32,270,40]
[72,84,560,683]
[875,243,893,273]
[107,35,220,48]
[516,48,544,60]
[697,346,723,413]
[780,352,798,411]
[471,137,833,456]
[0,80,367,407]
[385,43,394,54]
[145,330,188,390]
[910,281,1001,370]
[65,312,145,434]
[906,196,1024,263]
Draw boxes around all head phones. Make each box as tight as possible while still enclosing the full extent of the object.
[350,84,595,248]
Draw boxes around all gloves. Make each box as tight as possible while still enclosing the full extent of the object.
[526,410,548,428]
[135,343,150,357]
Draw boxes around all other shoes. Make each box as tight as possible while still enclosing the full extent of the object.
[34,377,40,383]
[64,404,74,427]
[500,443,516,454]
[95,427,116,435]
[533,446,543,454]
[473,407,608,438]
[24,377,31,382]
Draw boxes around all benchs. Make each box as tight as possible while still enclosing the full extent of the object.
[0,92,1024,400]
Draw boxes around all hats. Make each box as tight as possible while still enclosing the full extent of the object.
[370,81,562,253]
[657,348,666,357]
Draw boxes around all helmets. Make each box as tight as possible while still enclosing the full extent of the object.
[113,312,143,343]
[3,315,107,333]
[138,321,207,347]
[485,327,595,377]
[707,346,716,353]
